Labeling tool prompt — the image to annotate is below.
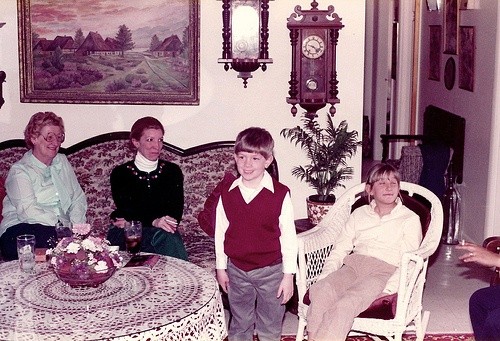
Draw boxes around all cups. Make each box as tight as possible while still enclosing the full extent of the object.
[54,221,73,241]
[124,221,142,259]
[16,235,35,272]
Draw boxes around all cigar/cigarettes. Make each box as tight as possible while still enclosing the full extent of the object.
[461,239,465,246]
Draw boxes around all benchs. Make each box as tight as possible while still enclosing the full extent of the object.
[0,131,280,308]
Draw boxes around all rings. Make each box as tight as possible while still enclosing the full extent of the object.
[470,252,473,257]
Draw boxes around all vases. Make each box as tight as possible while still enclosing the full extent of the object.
[51,255,122,289]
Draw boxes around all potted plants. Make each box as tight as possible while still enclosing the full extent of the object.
[279,110,368,227]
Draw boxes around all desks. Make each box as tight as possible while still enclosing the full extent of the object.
[0,251,228,341]
[286,218,317,316]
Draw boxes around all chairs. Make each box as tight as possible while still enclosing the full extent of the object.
[379,105,466,246]
[294,180,444,341]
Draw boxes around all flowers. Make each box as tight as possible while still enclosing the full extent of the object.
[44,223,124,270]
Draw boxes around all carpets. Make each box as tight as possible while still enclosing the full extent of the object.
[252,332,478,341]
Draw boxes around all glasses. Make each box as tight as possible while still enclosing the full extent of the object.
[35,132,65,143]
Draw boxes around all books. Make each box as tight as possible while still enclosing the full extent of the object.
[122,254,160,271]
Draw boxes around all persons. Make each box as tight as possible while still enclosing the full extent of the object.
[456,241,500,341]
[214,126,298,341]
[0,112,87,262]
[106,117,188,261]
[305,162,423,341]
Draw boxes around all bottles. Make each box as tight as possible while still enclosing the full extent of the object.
[441,175,460,246]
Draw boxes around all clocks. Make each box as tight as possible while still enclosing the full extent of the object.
[216,0,276,89]
[285,0,346,119]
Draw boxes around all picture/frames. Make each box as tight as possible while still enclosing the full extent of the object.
[428,24,443,83]
[458,26,476,93]
[16,0,200,106]
[443,0,462,56]
